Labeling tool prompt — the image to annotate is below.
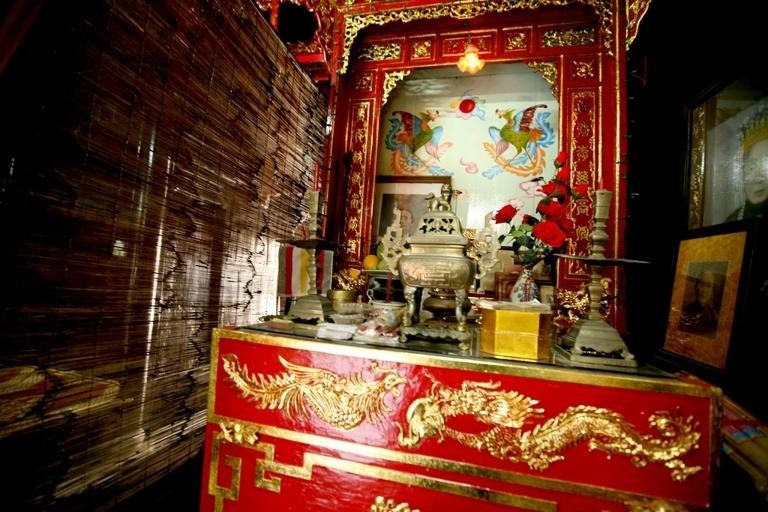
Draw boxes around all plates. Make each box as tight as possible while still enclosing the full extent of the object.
[361,270,398,282]
[330,301,370,325]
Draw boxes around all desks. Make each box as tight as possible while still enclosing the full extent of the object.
[194,313,724,512]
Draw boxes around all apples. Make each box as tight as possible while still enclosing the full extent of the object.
[376,244,398,270]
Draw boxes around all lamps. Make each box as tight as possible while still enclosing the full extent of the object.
[454,33,486,76]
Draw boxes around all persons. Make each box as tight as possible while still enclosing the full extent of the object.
[722,110,768,222]
[676,271,719,334]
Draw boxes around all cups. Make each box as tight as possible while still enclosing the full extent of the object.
[373,300,406,327]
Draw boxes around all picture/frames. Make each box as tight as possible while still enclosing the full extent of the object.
[649,227,753,382]
[367,171,455,257]
[677,53,767,242]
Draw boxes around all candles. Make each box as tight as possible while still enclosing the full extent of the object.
[312,162,320,191]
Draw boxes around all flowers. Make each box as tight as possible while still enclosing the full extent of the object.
[488,150,588,271]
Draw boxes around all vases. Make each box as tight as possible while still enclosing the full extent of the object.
[507,261,542,304]
